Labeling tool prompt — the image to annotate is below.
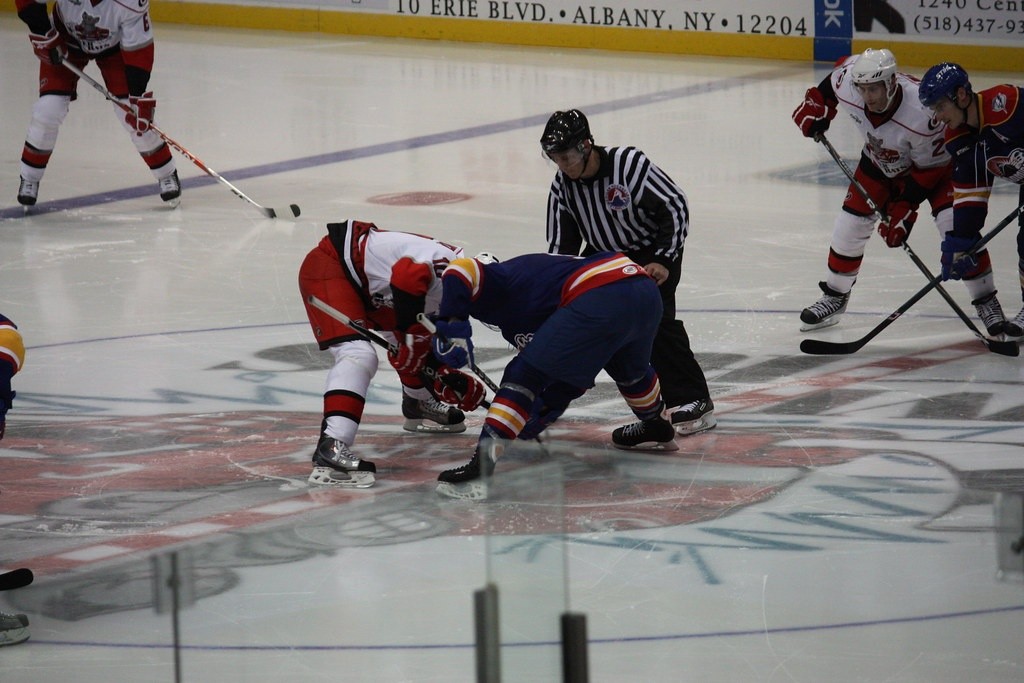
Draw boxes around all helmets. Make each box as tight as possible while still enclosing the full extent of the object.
[920,63,968,105]
[851,48,896,86]
[475,252,500,266]
[540,110,590,151]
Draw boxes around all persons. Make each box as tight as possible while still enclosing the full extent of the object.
[0,314,32,647]
[14,0,182,208]
[298,109,718,485]
[791,47,1024,337]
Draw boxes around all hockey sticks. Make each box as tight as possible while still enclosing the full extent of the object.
[817,126,1020,359]
[59,57,301,220]
[416,310,500,395]
[304,292,492,411]
[799,210,1018,356]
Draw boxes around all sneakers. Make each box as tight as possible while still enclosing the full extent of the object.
[436,450,496,499]
[403,389,466,432]
[1006,308,1023,343]
[611,405,680,452]
[308,434,378,486]
[800,281,856,331]
[17,174,40,212]
[0,613,30,647]
[970,290,1006,342]
[671,397,717,436]
[159,168,181,209]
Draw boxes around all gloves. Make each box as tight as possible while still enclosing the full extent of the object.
[125,91,156,135]
[941,229,982,280]
[433,366,487,411]
[878,201,917,247]
[792,86,837,139]
[386,324,433,375]
[28,26,69,64]
[433,322,473,369]
